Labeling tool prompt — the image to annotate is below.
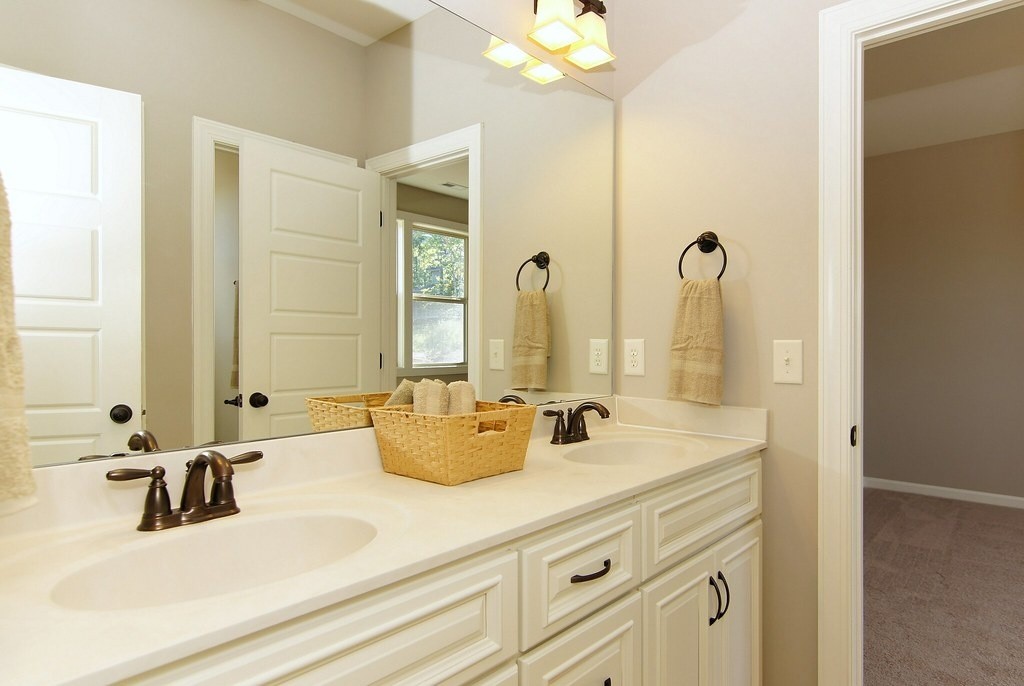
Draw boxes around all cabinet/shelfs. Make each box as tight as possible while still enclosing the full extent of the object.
[0,420,765,686]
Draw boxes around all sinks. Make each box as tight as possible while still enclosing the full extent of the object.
[53,507,379,614]
[564,435,705,471]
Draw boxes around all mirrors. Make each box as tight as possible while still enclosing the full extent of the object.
[0,0,619,468]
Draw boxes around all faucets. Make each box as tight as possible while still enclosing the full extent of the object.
[125,428,162,453]
[568,403,609,443]
[500,394,527,405]
[176,449,236,515]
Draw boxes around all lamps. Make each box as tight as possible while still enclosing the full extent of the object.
[482,35,565,85]
[526,0,618,71]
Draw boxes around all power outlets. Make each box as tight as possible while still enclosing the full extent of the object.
[624,339,647,376]
[588,338,608,374]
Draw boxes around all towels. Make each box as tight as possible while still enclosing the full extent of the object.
[384,378,414,407]
[510,291,555,391]
[1,184,33,496]
[666,280,724,406]
[414,380,480,420]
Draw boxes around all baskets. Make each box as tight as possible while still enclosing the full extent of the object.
[305,391,393,433]
[369,400,537,486]
